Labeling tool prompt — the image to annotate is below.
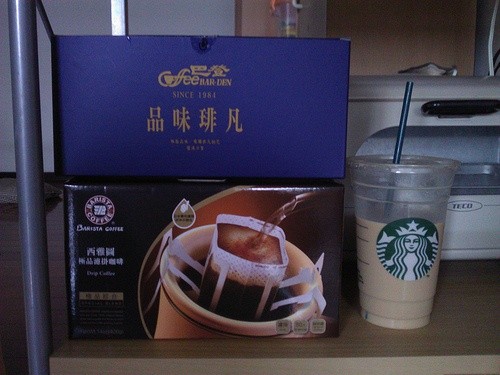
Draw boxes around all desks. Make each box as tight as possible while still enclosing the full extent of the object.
[48,259,500,375]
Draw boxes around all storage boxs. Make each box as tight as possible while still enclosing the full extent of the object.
[63,176,345,341]
[50,35,350,180]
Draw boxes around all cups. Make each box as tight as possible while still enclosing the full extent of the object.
[351,155,459,329]
[154,224,323,339]
